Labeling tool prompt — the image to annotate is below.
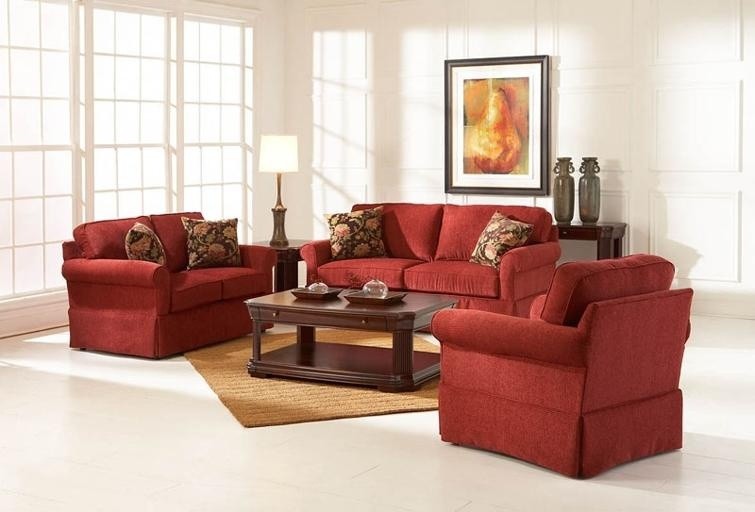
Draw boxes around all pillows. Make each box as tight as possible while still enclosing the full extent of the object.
[541,252,676,325]
[124,221,166,268]
[322,205,390,262]
[180,216,242,272]
[468,210,536,273]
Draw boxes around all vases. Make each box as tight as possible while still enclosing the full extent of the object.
[552,156,576,227]
[578,156,601,227]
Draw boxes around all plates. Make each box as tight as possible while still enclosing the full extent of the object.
[343,291,408,305]
[291,288,343,300]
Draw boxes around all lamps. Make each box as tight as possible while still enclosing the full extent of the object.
[258,134,300,248]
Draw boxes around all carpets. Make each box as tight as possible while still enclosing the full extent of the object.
[181,327,442,427]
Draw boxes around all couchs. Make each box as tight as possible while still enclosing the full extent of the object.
[429,253,693,482]
[61,211,279,361]
[299,203,562,332]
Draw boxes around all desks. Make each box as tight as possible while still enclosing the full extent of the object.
[548,222,627,262]
[251,239,314,293]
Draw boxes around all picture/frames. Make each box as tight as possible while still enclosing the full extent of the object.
[443,55,552,197]
